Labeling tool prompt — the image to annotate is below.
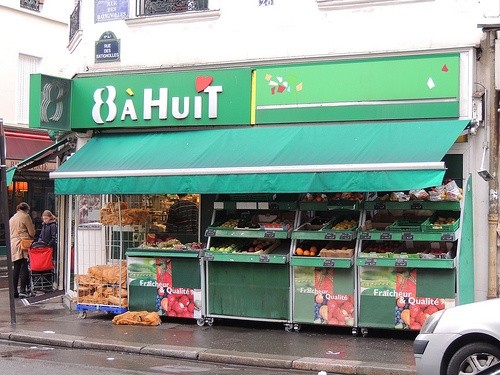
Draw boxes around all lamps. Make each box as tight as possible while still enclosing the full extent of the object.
[8,180,28,191]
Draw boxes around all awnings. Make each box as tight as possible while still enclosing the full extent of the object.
[5,119,470,195]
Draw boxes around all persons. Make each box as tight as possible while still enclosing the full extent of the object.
[9,202,58,299]
[165,194,199,245]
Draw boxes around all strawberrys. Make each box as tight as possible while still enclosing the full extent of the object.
[316,293,355,326]
[156,287,195,318]
[397,297,446,329]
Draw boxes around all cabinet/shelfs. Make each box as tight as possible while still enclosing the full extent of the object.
[126,244,206,327]
[358,175,474,338]
[204,194,291,332]
[291,192,357,335]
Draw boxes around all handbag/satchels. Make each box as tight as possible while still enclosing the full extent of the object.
[21,239,34,251]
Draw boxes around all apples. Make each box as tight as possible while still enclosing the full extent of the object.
[247,239,271,252]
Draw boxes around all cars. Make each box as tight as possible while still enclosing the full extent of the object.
[412,298,500,375]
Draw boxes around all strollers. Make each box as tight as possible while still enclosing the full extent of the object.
[26,241,53,294]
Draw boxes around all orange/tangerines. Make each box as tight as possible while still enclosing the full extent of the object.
[296,246,317,255]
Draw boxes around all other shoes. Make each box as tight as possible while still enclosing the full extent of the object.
[14,291,31,298]
